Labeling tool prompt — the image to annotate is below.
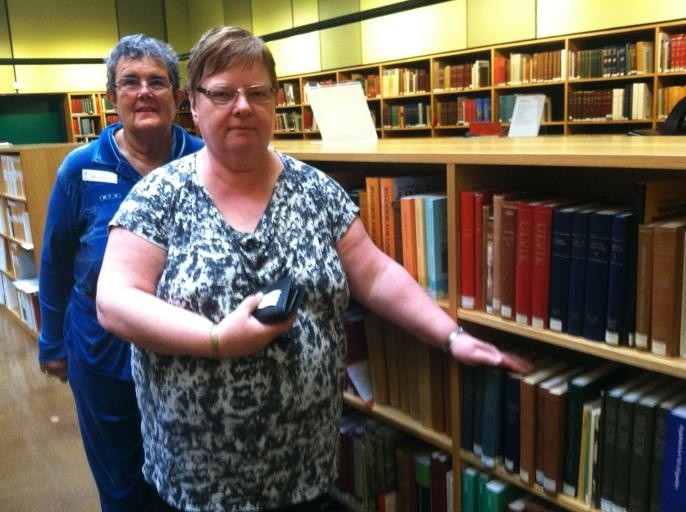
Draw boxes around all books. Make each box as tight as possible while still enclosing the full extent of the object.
[72,93,120,143]
[273,31,686,131]
[1,156,38,332]
[350,177,684,360]
[172,93,202,140]
[319,362,686,512]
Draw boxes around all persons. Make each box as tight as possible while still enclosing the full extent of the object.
[40,34,206,512]
[94,29,537,512]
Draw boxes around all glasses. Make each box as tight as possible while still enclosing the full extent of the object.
[114,78,173,93]
[195,83,274,103]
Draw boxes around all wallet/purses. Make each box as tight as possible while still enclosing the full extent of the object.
[251,274,312,322]
